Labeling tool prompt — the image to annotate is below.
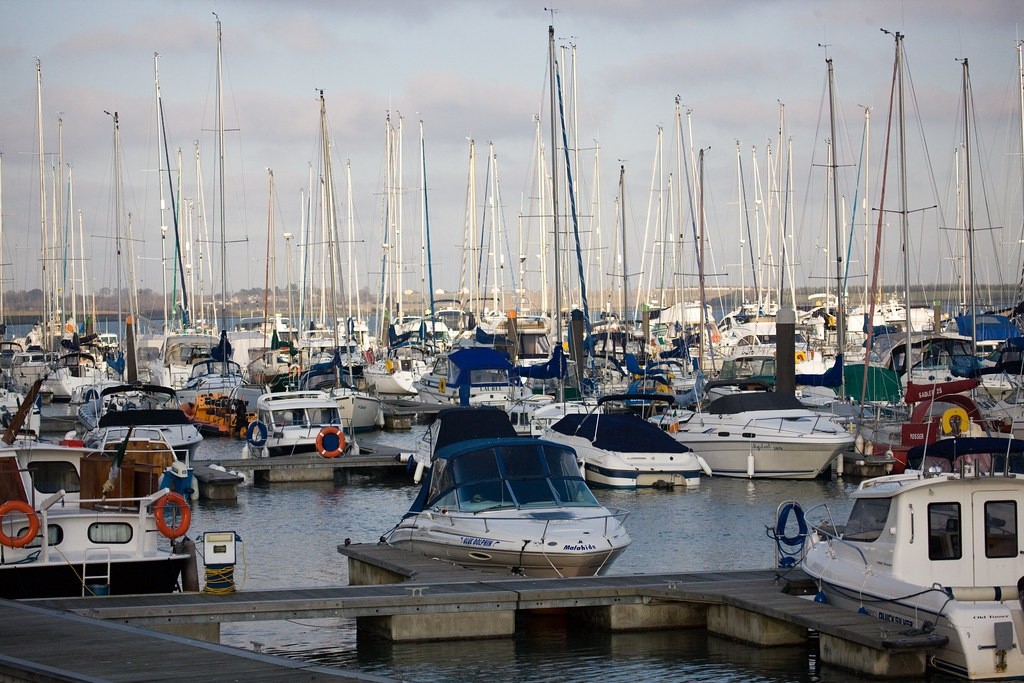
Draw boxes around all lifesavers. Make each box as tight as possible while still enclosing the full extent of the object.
[655,384,669,403]
[384,359,392,372]
[828,314,835,326]
[795,350,808,364]
[941,407,970,435]
[246,421,267,446]
[0,500,40,548]
[315,427,346,458]
[59,439,84,447]
[154,490,192,539]
[438,377,447,393]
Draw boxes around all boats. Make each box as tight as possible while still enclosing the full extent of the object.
[244,388,359,460]
[652,377,855,478]
[0,427,191,601]
[539,395,712,489]
[800,350,1024,683]
[405,407,520,484]
[389,437,633,578]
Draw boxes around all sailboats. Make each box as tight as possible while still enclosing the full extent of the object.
[0,7,1024,438]
